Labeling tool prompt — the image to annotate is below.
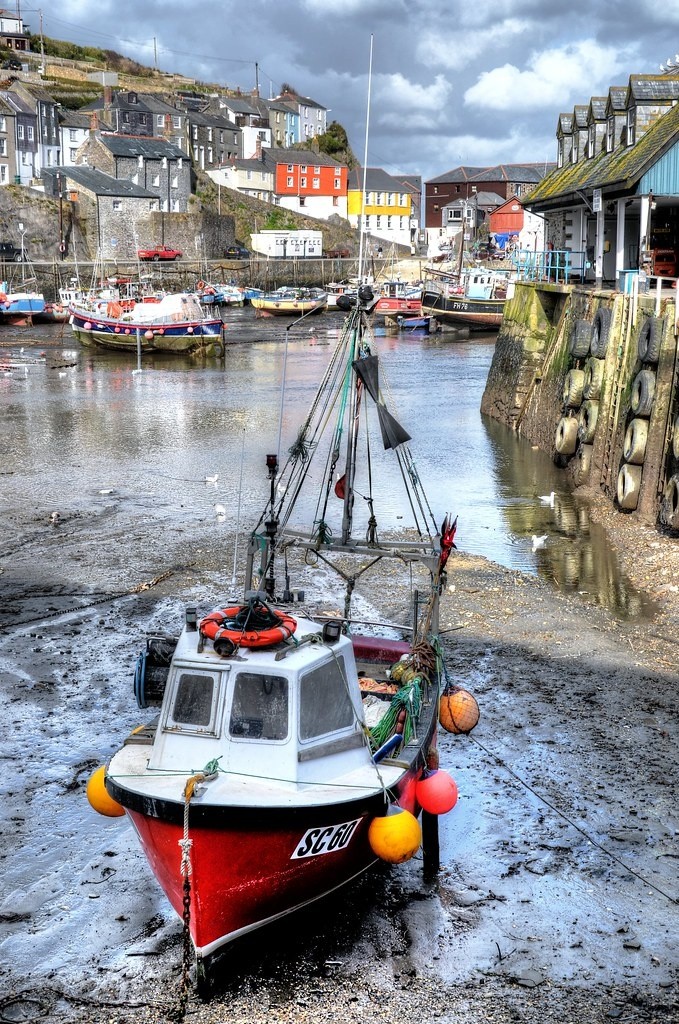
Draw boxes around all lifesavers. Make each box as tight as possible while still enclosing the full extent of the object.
[197,281,204,289]
[119,296,135,314]
[51,302,63,313]
[200,606,297,647]
[107,301,123,318]
[555,308,679,528]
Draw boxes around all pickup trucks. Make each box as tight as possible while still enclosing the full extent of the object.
[138,245,184,262]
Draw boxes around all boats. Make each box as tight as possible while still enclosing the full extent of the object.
[104,34,458,986]
[0,240,511,359]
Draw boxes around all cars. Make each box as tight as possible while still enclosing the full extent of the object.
[0,242,27,262]
[226,246,250,260]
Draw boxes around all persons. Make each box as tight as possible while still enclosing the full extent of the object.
[378,245,383,259]
